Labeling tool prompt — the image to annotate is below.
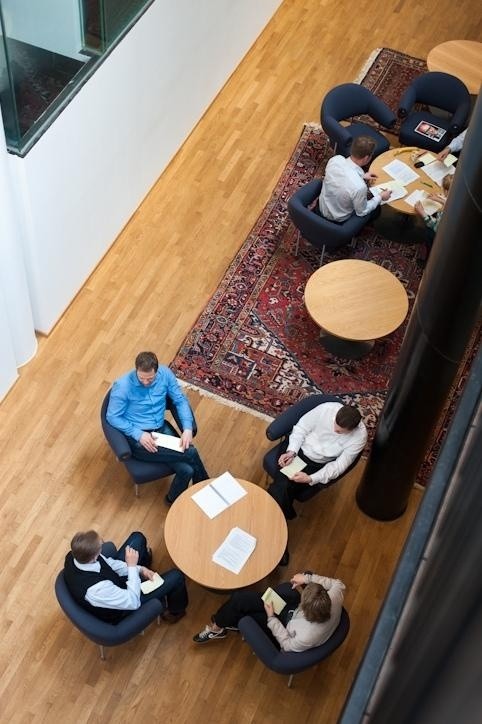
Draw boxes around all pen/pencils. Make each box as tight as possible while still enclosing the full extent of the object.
[210,484,230,505]
[421,182,433,188]
[381,188,386,191]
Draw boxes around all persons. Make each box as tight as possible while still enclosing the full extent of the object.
[64,530,188,623]
[106,351,210,506]
[193,573,346,645]
[315,136,391,226]
[415,174,454,269]
[266,402,368,566]
[437,128,468,161]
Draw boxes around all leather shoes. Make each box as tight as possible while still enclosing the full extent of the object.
[280,548,289,566]
[161,608,185,622]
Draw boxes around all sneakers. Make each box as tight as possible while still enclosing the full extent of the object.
[193,625,228,644]
[211,615,240,631]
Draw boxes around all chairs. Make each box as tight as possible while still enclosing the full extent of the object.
[286,177,372,271]
[53,568,165,660]
[238,606,351,689]
[396,69,469,154]
[321,83,398,172]
[101,383,199,497]
[261,393,366,505]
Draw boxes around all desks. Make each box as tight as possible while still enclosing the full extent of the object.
[301,254,410,362]
[367,145,457,245]
[161,476,291,591]
[426,39,482,95]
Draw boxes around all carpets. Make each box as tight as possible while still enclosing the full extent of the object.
[166,123,479,492]
[343,47,429,138]
